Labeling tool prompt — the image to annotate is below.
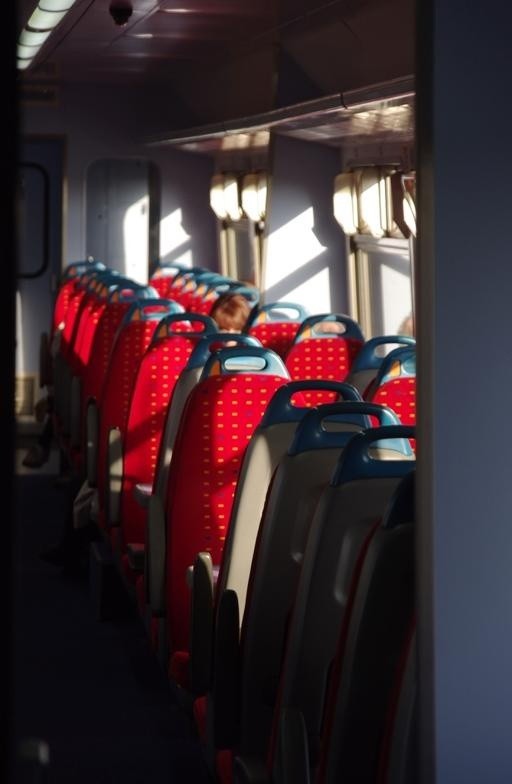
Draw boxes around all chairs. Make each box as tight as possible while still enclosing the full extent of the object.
[39,259,424,783]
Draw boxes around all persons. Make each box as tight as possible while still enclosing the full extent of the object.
[211,294,252,347]
